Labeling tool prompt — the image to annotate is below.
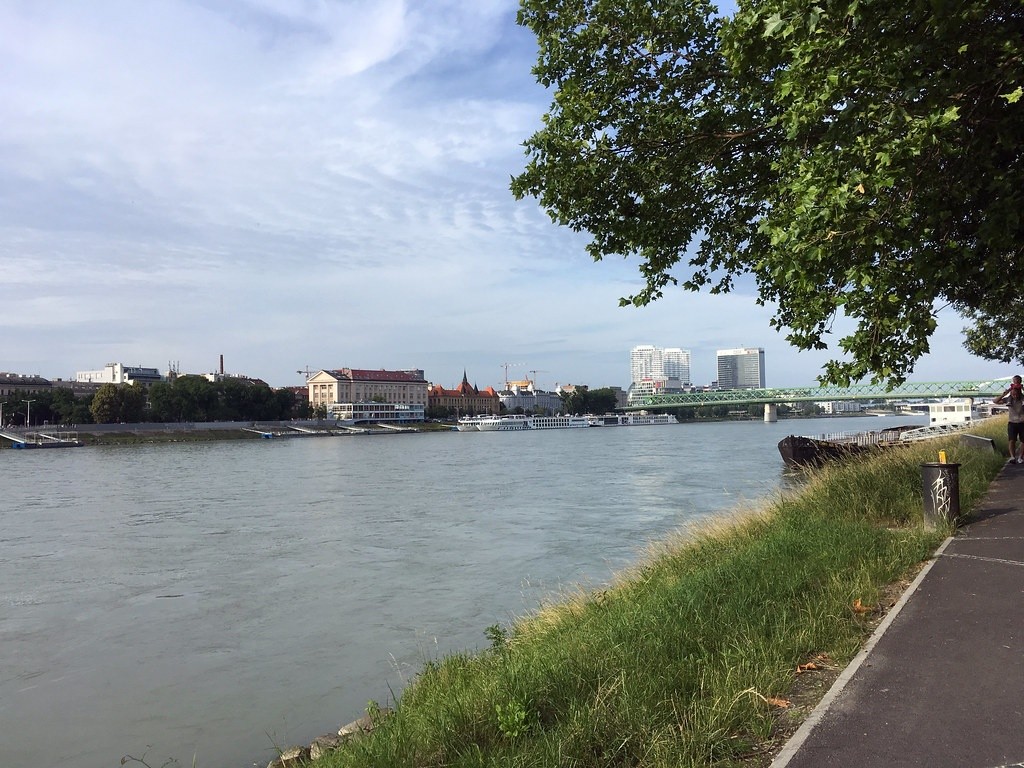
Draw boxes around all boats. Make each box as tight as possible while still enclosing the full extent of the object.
[454,411,680,432]
[777,434,871,471]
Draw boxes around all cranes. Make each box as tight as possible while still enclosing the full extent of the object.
[296,365,320,386]
[499,361,551,391]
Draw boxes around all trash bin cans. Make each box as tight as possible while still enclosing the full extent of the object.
[919,462,963,531]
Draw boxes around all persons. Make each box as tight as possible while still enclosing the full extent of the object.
[993,387,1024,463]
[1003,376,1024,407]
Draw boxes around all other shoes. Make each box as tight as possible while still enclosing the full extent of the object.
[1010,458,1016,463]
[1018,458,1023,463]
[1006,402,1011,406]
[1022,401,1024,406]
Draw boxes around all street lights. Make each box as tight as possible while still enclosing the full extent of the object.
[22,399,36,430]
[0,402,8,428]
